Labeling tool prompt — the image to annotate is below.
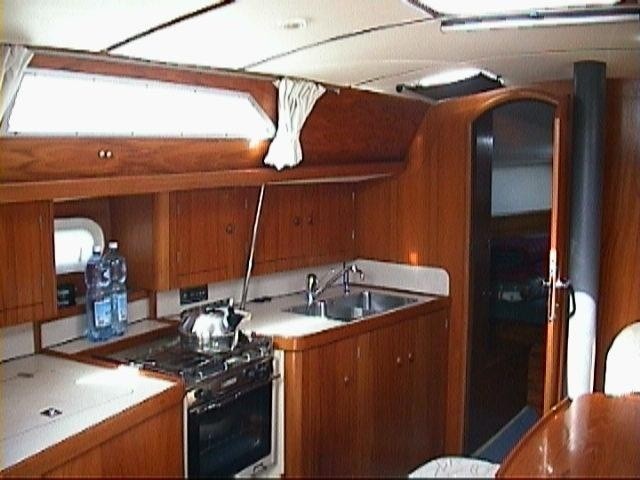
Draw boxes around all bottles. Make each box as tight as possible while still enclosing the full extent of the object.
[84,242,127,343]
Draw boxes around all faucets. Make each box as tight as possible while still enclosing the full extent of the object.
[306,265,365,303]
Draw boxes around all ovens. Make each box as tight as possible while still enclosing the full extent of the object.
[187,356,278,480]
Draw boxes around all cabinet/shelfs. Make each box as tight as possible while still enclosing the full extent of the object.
[286,332,373,480]
[0,202,58,323]
[171,192,244,289]
[245,183,354,273]
[373,312,449,479]
[44,406,183,480]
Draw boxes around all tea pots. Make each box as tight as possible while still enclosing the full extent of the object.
[179,296,253,357]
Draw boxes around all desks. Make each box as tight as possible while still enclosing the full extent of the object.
[496,395,640,480]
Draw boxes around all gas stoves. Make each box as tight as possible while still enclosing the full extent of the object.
[109,328,274,379]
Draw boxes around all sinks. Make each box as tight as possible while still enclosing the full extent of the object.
[284,289,420,323]
